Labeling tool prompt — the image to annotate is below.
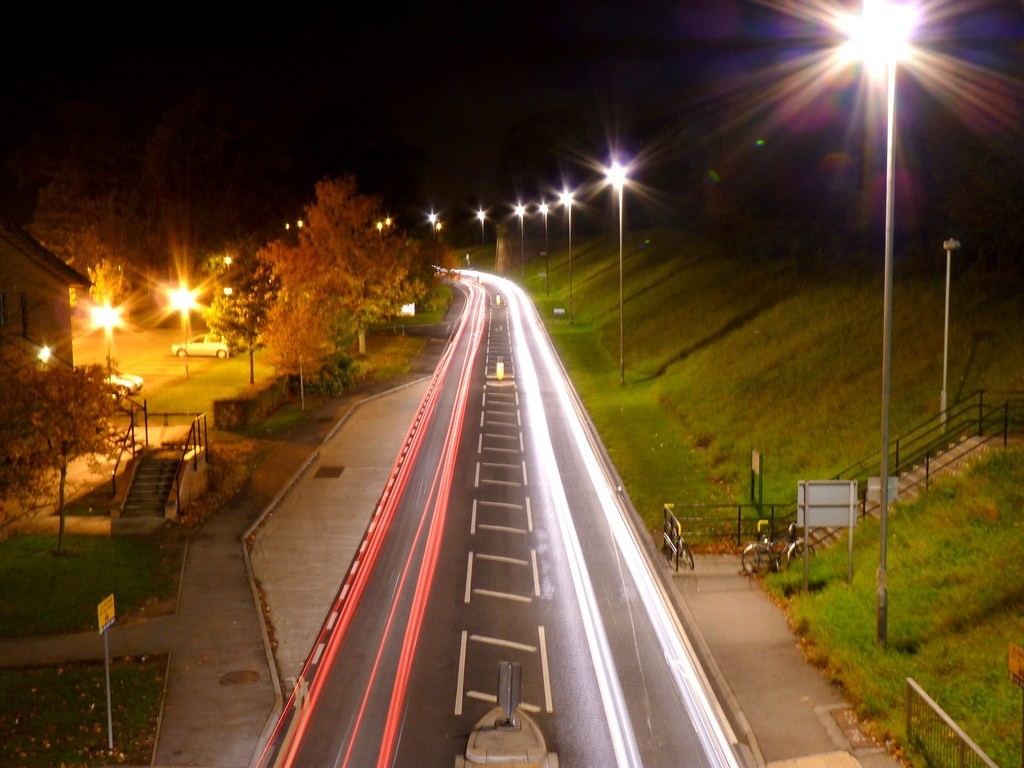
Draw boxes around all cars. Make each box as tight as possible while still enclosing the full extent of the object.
[100,368,144,396]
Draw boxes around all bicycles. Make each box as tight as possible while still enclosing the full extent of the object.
[667,522,694,570]
[740,521,815,577]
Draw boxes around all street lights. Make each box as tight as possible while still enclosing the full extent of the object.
[539,204,549,297]
[515,206,524,285]
[560,192,573,325]
[847,2,914,648]
[607,162,626,387]
[175,289,191,378]
[101,306,116,374]
[477,211,485,273]
[429,213,437,235]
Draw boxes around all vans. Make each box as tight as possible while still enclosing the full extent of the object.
[172,332,233,358]
